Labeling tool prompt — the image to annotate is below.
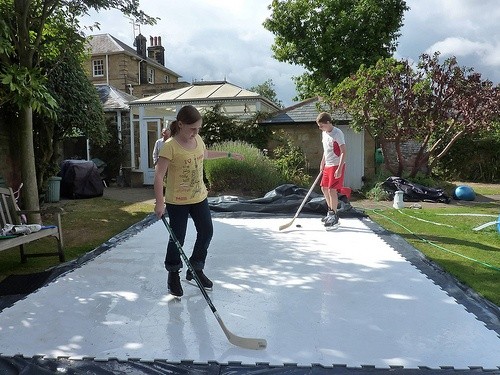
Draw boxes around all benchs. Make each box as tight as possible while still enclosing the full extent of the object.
[0,187,66,263]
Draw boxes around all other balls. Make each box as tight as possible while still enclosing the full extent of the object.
[295,225,301,228]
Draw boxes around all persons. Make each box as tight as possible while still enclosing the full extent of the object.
[316,112,345,227]
[154,106,213,297]
[152,128,170,164]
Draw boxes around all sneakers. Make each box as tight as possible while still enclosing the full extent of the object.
[324,210,340,231]
[321,207,331,225]
[186,266,214,291]
[167,271,183,301]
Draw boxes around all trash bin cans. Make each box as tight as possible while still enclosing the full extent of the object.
[45,176,62,203]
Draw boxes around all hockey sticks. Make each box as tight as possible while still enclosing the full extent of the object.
[279,172,322,231]
[160,216,267,352]
